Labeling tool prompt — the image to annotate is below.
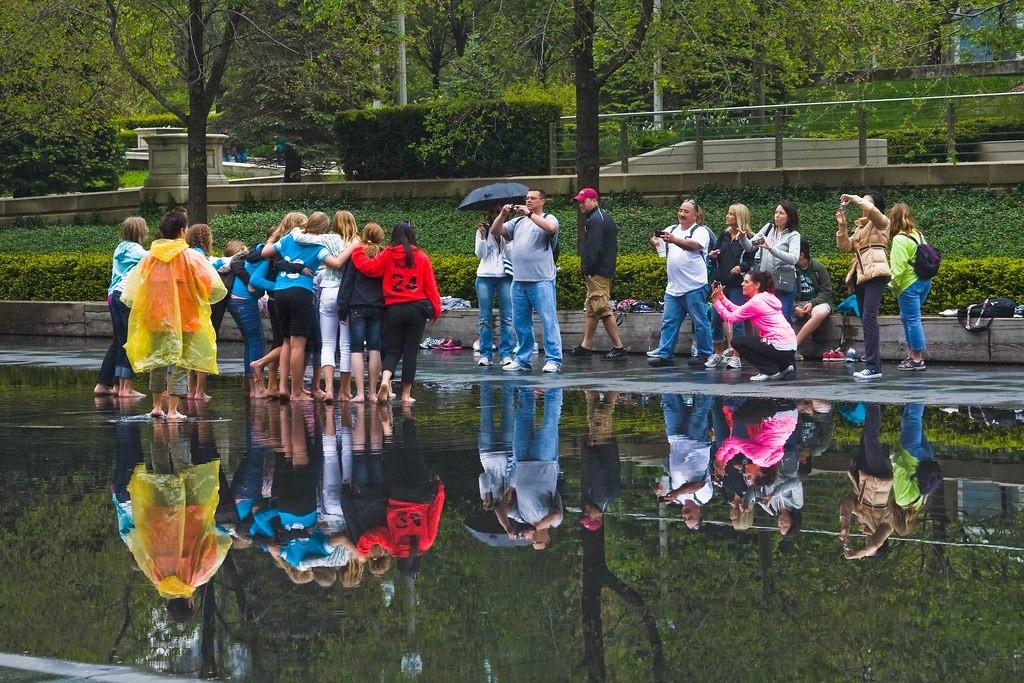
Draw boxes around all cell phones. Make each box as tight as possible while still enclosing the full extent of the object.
[658,495,670,502]
[655,231,665,237]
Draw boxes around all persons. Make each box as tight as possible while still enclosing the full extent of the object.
[94,208,440,400]
[495,385,564,552]
[660,393,835,540]
[474,201,514,366]
[890,403,933,536]
[835,191,892,378]
[274,135,286,165]
[580,390,620,530]
[490,189,562,374]
[646,200,831,381]
[889,203,932,369]
[93,394,442,625]
[222,128,232,161]
[565,188,627,361]
[232,137,247,162]
[479,380,515,510]
[838,400,892,559]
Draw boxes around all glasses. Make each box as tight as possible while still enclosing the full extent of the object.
[684,198,696,205]
[523,196,544,202]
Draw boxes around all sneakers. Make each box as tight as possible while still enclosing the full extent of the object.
[646,347,674,366]
[542,362,560,372]
[897,355,926,371]
[688,346,741,369]
[1013,304,1024,317]
[633,305,655,313]
[749,365,795,381]
[419,337,539,354]
[478,357,531,371]
[823,347,882,379]
[563,345,627,360]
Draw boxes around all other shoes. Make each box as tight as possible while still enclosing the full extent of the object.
[795,345,804,360]
[938,308,959,317]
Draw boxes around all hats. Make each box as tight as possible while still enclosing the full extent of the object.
[579,516,603,531]
[574,188,598,202]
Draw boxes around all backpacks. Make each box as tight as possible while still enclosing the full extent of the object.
[896,228,941,279]
[665,225,718,282]
[958,295,1015,368]
[513,213,559,264]
[903,459,941,512]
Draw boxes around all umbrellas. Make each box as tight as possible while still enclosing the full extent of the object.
[462,507,534,548]
[458,182,530,213]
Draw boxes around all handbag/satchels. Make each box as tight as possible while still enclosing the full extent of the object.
[616,299,635,326]
[774,266,795,292]
[503,257,514,279]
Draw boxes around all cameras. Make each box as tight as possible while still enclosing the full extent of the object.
[714,281,721,288]
[488,503,498,510]
[839,202,845,213]
[510,207,518,213]
[713,249,722,255]
[751,239,762,246]
[483,222,492,228]
[842,540,850,551]
[715,476,723,483]
[514,531,526,540]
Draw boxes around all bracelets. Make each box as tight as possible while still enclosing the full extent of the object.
[528,210,535,217]
[532,525,537,534]
